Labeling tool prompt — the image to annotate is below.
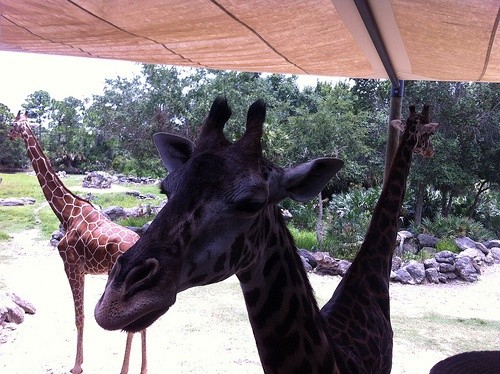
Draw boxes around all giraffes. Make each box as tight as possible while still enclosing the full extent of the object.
[8,109,149,374]
[320,104,439,374]
[93,95,342,374]
